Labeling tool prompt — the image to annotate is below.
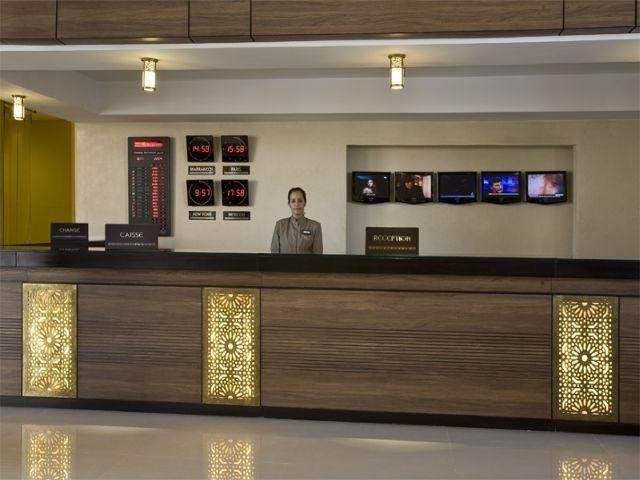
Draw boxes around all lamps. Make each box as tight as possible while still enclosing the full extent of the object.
[387,54,407,92]
[10,94,27,123]
[141,57,159,93]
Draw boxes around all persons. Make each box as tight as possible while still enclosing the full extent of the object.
[528,174,564,194]
[271,187,323,254]
[488,178,503,193]
[400,178,425,201]
[363,179,374,194]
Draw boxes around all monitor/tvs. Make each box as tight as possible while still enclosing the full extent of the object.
[393,171,434,205]
[481,170,522,205]
[436,171,478,205]
[525,170,567,204]
[351,171,391,204]
